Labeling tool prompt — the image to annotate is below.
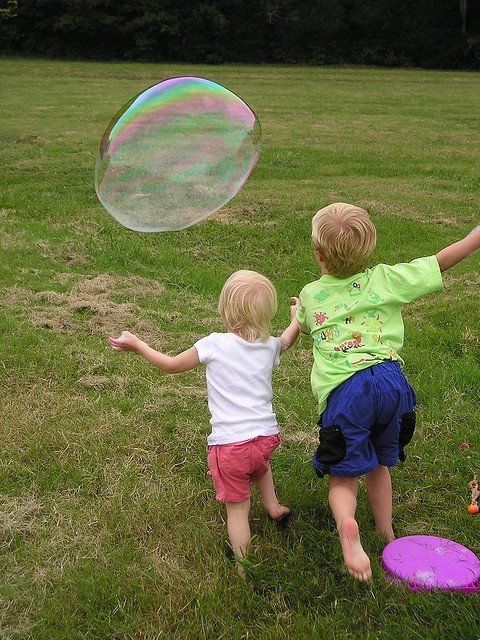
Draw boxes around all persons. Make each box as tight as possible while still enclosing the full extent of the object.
[108,269,301,582]
[297,204,480,580]
